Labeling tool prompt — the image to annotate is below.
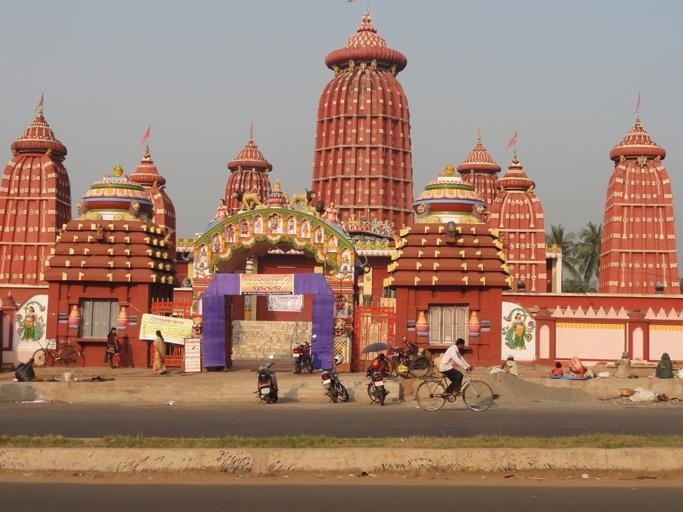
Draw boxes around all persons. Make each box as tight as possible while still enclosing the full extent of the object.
[614,352,631,378]
[152,330,168,374]
[569,356,587,376]
[656,353,673,378]
[550,362,562,376]
[437,338,473,402]
[198,199,392,281]
[106,325,120,352]
[503,355,518,377]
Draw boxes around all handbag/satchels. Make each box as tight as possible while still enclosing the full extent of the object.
[113,353,120,368]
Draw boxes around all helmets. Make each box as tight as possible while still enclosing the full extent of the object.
[333,354,344,365]
[371,359,381,369]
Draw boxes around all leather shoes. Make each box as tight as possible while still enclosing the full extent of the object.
[453,391,465,396]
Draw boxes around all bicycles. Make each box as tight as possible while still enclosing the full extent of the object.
[416,367,494,412]
[401,337,430,378]
[33,337,85,367]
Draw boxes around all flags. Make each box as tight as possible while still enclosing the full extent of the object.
[634,94,640,109]
[141,126,151,143]
[34,94,44,111]
[508,132,518,148]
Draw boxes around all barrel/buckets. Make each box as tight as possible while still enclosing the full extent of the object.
[62,369,74,382]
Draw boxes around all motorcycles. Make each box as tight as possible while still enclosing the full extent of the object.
[312,356,349,403]
[106,344,115,369]
[389,348,411,377]
[250,355,278,403]
[293,335,317,374]
[366,360,390,405]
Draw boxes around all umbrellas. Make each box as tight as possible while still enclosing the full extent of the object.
[361,342,392,356]
